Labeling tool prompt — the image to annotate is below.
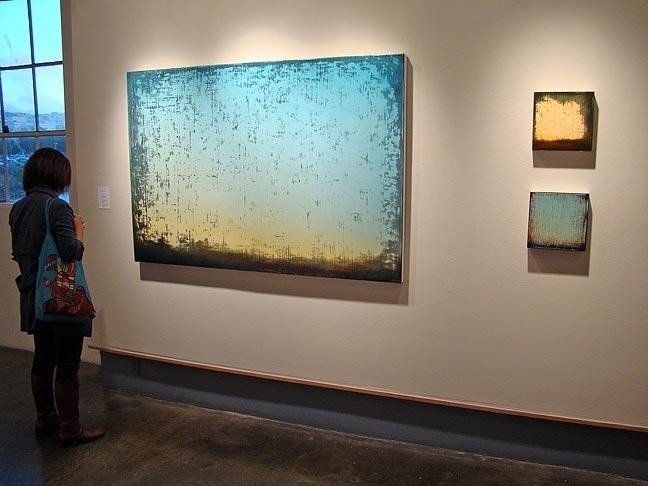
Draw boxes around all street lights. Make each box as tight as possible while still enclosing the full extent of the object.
[33,198,98,325]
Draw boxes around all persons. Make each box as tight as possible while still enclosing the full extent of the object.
[9,146,106,448]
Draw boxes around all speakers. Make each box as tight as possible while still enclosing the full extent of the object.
[33,377,107,446]
